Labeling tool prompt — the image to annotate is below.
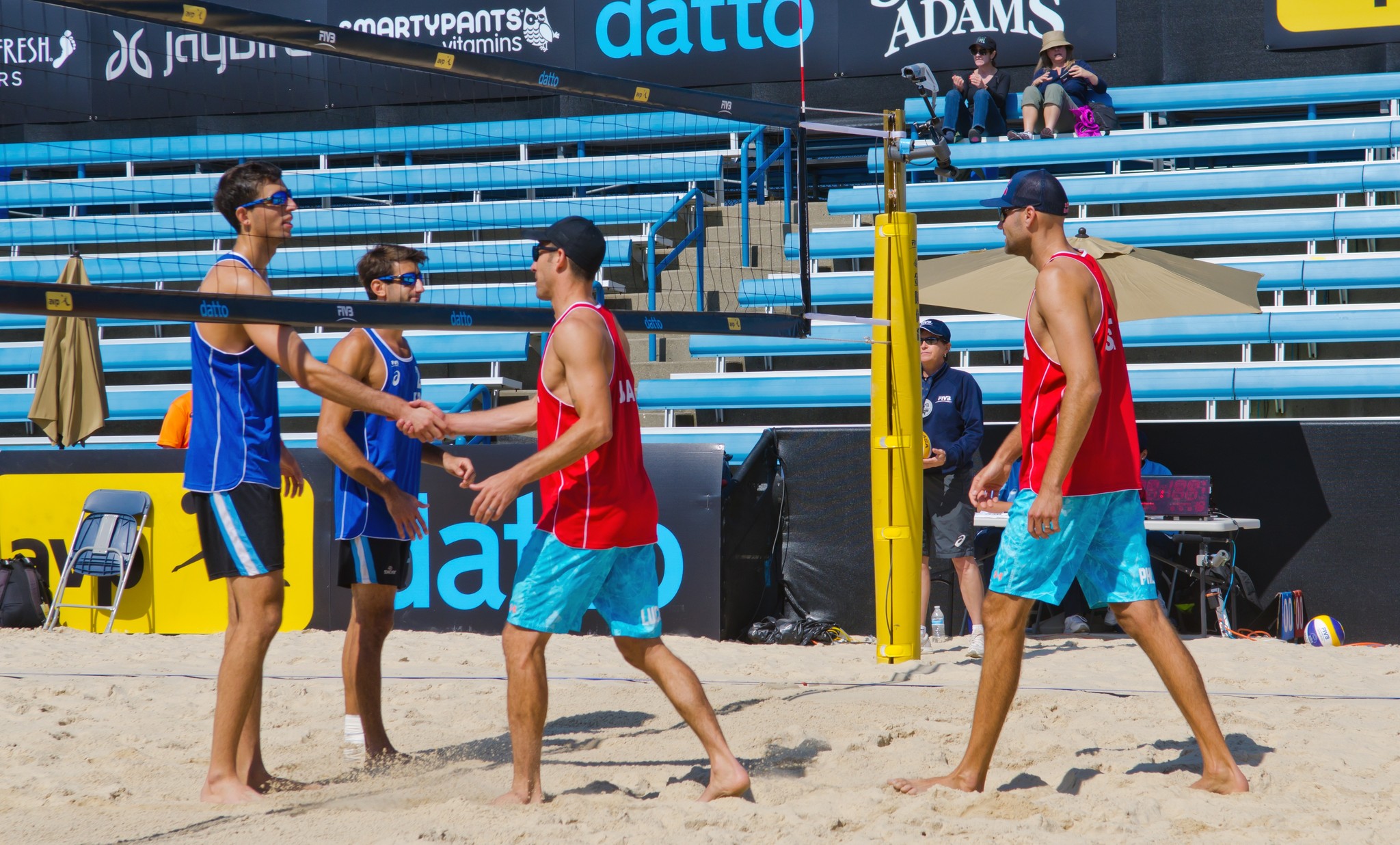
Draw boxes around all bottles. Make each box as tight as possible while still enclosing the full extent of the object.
[931,605,945,643]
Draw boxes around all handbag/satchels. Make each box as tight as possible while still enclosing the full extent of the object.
[1086,101,1122,131]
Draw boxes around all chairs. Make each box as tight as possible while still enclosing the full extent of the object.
[43,489,151,633]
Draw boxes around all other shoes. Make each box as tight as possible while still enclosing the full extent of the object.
[1104,606,1118,625]
[1157,590,1168,617]
[968,128,982,143]
[931,131,954,144]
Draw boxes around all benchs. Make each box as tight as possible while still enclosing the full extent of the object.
[629,72,1400,423]
[0,111,766,453]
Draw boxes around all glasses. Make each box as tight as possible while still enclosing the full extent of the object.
[920,337,949,345]
[235,188,293,211]
[376,272,423,286]
[532,245,558,261]
[969,47,990,56]
[998,203,1042,223]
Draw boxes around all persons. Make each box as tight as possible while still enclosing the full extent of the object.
[157,389,192,449]
[917,319,986,658]
[397,215,751,805]
[973,440,1181,636]
[940,36,1011,144]
[1007,31,1107,141]
[890,168,1249,796]
[182,159,454,805]
[317,245,475,764]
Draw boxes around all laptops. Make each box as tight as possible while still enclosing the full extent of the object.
[1140,474,1216,517]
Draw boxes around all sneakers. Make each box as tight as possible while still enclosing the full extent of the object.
[1040,127,1054,139]
[919,633,935,654]
[965,628,985,658]
[1063,614,1090,634]
[1007,130,1033,141]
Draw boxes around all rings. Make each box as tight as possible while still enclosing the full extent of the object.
[1044,524,1051,529]
[489,507,495,513]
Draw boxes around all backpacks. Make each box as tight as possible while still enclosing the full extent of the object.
[0,553,60,628]
[1196,563,1264,639]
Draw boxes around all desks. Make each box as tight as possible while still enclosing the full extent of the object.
[973,517,1260,639]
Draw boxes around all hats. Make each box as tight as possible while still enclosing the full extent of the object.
[1039,30,1074,57]
[523,215,606,274]
[920,319,951,340]
[969,35,996,51]
[980,168,1069,216]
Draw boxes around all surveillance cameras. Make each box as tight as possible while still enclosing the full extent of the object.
[903,65,921,79]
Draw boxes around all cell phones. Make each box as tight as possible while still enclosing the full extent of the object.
[1061,71,1074,84]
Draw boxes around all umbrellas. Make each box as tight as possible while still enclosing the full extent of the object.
[917,228,1264,323]
[26,248,106,448]
